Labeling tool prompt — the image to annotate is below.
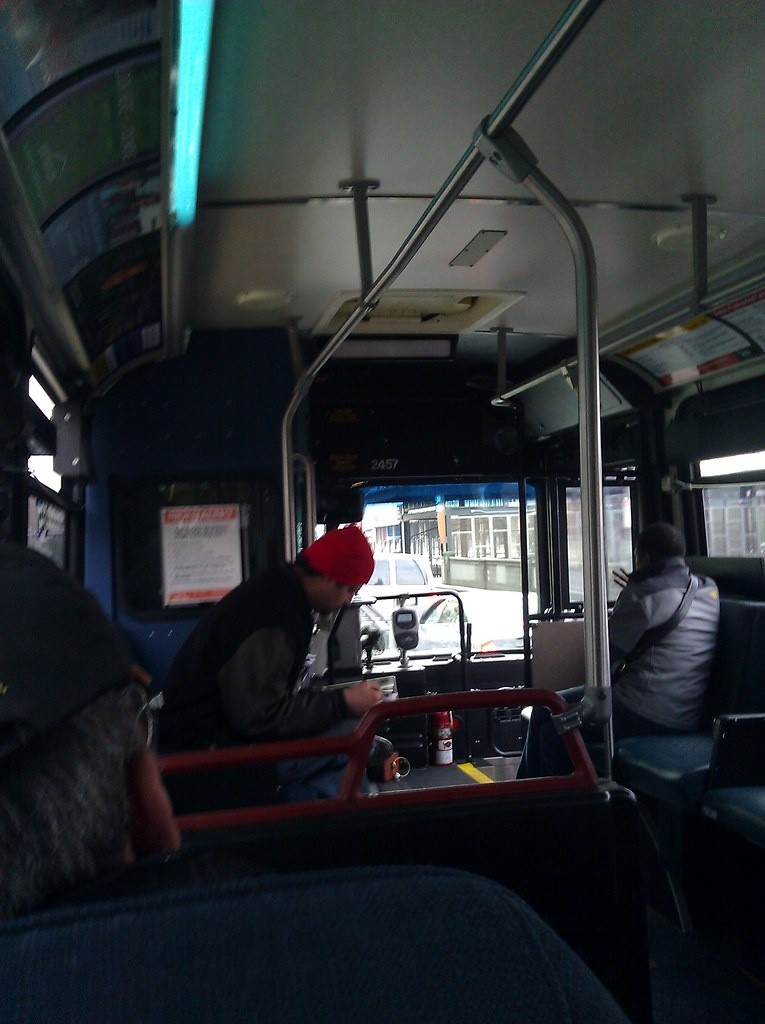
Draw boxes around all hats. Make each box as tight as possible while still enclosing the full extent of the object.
[298,525,374,586]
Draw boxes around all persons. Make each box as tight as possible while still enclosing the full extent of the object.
[0,542,187,924]
[154,521,384,796]
[516,522,722,780]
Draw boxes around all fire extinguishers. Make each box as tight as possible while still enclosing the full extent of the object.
[433,711,460,767]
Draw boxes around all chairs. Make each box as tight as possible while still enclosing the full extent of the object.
[537,597,764,932]
